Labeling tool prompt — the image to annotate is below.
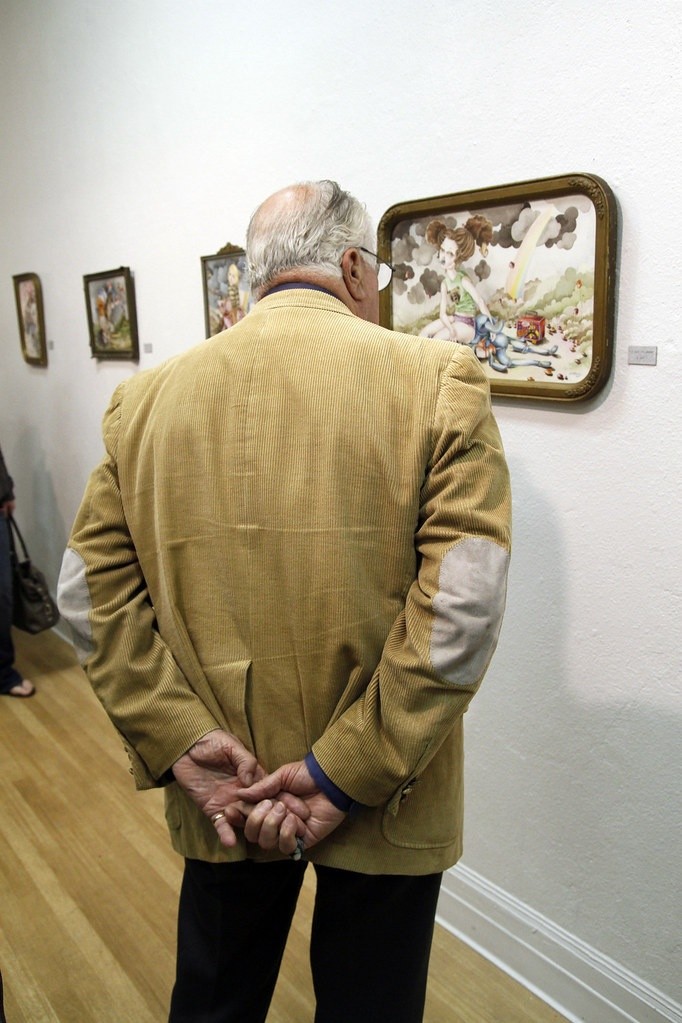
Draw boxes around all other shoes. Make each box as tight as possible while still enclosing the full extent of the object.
[1,678,37,698]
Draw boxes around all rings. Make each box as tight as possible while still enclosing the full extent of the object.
[210,812,225,825]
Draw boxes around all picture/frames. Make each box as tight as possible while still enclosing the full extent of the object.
[11,272,49,369]
[200,243,253,340]
[374,172,620,405]
[83,266,140,363]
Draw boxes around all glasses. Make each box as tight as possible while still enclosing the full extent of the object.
[356,247,395,292]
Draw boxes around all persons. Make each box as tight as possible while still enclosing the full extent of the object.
[54,178,512,1023]
[0,444,40,698]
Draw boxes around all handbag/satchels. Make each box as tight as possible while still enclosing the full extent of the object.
[7,507,59,636]
